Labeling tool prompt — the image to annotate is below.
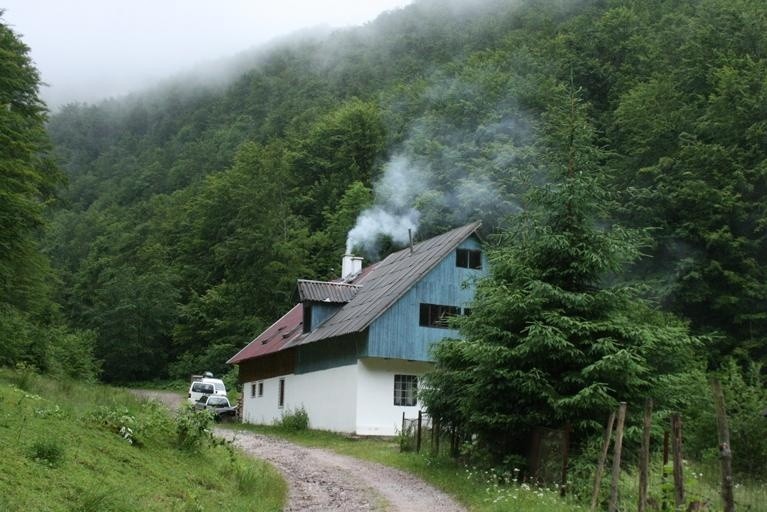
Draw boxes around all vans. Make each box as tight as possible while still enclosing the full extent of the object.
[201,377,227,399]
[187,381,216,409]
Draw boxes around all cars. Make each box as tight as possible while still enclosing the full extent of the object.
[213,406,239,423]
[194,396,231,417]
